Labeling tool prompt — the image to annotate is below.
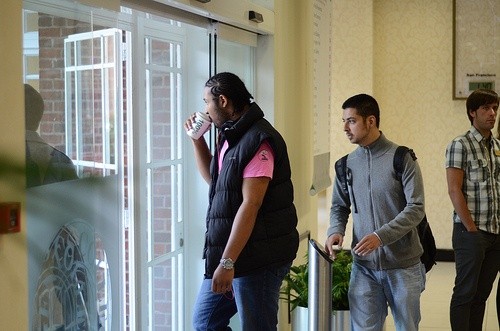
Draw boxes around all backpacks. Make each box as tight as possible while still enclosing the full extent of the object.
[335,146,438,273]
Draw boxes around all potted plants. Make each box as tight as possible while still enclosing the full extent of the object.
[279,250,352,331]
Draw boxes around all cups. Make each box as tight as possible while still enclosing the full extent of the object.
[186,111,211,140]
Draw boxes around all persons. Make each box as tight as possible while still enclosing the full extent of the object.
[444,88,500,331]
[183,72,300,331]
[324,94,426,331]
[24,82,102,331]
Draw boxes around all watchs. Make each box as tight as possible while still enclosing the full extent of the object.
[220,258,236,270]
